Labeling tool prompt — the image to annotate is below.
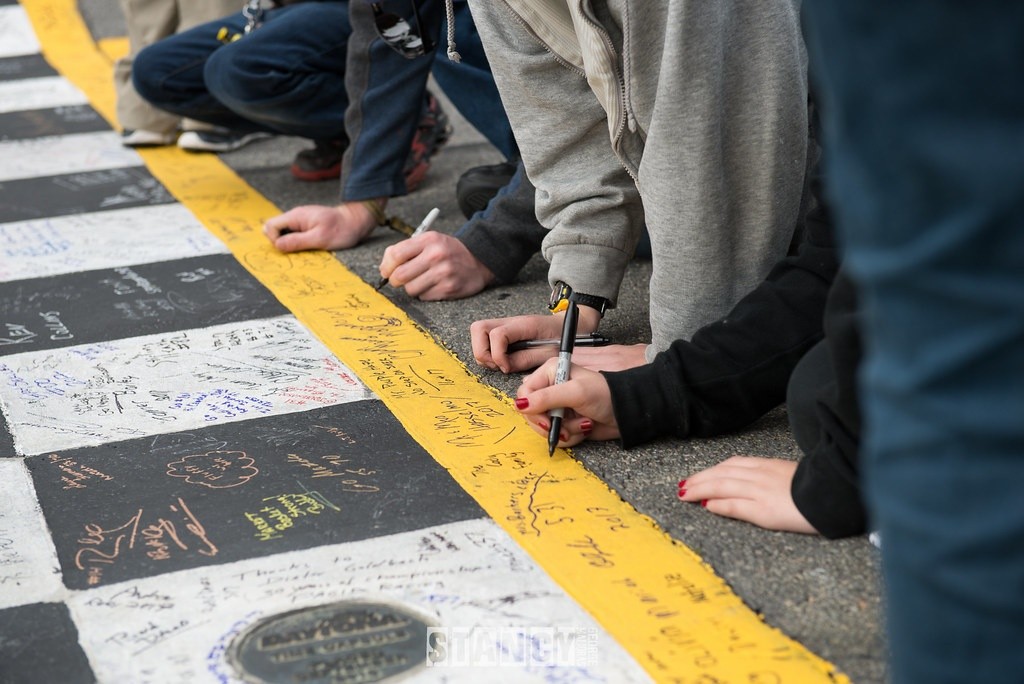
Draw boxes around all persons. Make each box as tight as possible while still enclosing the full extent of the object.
[114,0,1024,683]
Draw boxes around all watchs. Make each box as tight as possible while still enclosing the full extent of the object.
[549,281,606,316]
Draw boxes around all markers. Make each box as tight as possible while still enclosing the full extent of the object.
[375,206,441,291]
[547,298,582,458]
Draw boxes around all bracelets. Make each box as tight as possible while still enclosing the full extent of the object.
[364,200,414,236]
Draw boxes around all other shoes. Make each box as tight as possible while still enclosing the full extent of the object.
[457,163,519,222]
[401,90,452,192]
[177,127,275,150]
[293,149,344,181]
[121,128,177,144]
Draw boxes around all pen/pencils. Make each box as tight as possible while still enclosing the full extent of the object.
[508,331,611,351]
